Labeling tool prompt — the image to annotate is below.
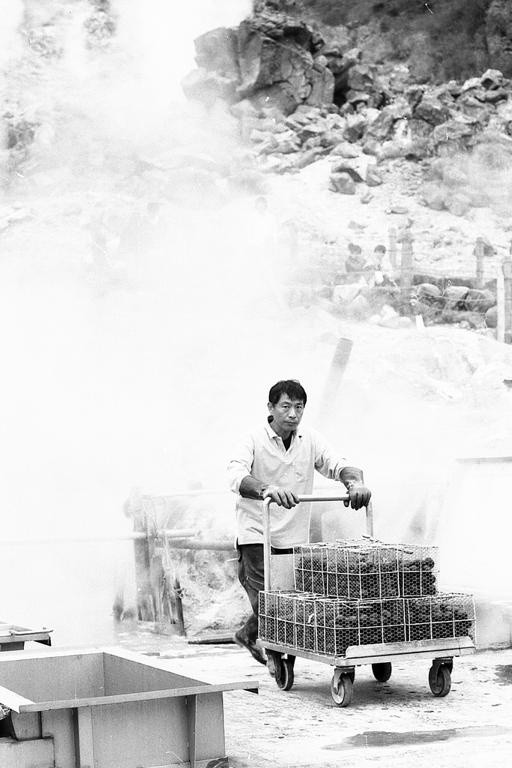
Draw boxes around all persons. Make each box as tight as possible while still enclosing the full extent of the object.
[345,243,366,272]
[229,380,371,666]
[363,245,393,270]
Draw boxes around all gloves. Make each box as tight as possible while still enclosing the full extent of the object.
[240,475,300,509]
[340,467,371,510]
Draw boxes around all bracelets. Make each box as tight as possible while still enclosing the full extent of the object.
[255,483,268,498]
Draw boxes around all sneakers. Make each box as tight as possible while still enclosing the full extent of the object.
[235,626,268,665]
[267,655,276,678]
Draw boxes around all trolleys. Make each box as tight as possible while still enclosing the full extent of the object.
[256,495,477,706]
